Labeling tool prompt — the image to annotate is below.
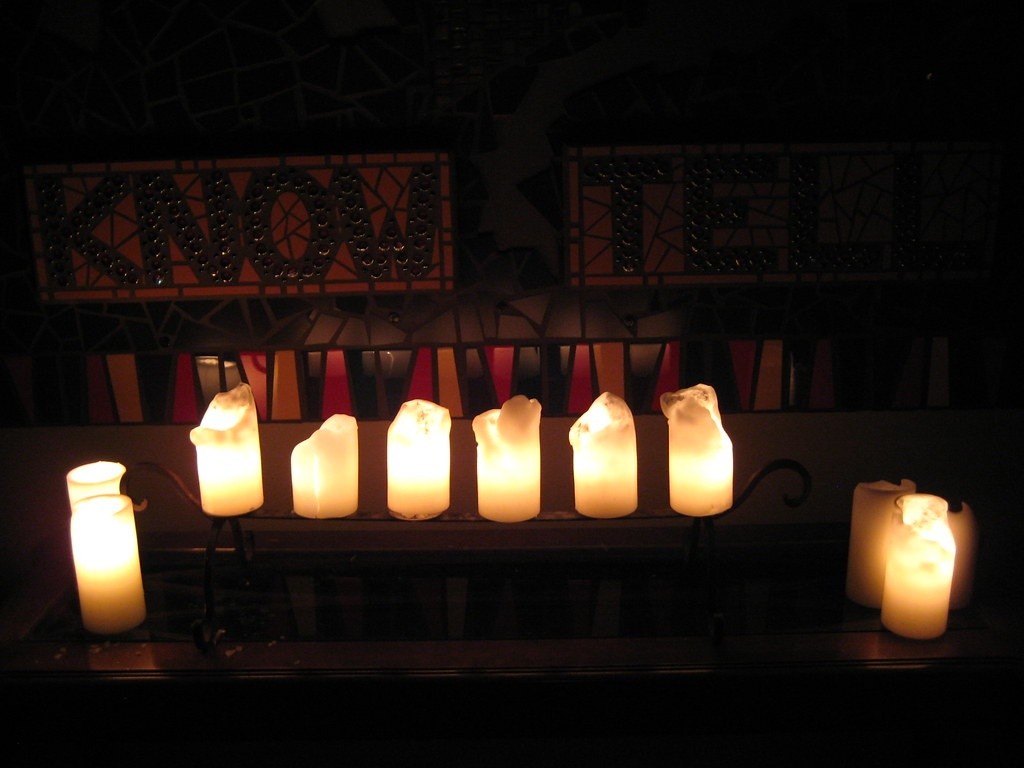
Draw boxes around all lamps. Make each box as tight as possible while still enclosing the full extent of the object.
[472,394,543,522]
[846,479,916,608]
[569,392,638,519]
[69,495,148,636]
[880,493,956,640]
[386,400,453,522]
[66,460,127,512]
[189,383,264,517]
[949,502,978,610]
[291,413,359,519]
[659,384,734,517]
